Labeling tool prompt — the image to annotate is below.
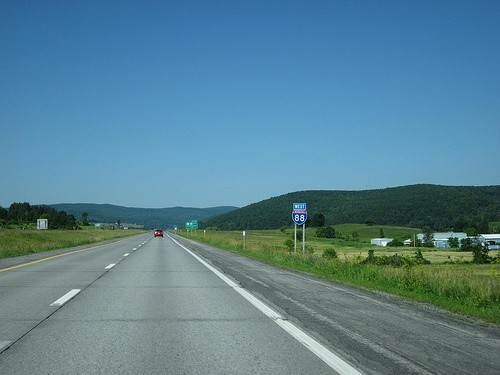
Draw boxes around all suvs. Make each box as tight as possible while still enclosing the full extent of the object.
[154,229,164,237]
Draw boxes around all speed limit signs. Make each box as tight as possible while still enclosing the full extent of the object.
[292,210,308,225]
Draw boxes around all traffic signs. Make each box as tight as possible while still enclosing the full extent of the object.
[185,220,199,229]
[293,203,306,211]
